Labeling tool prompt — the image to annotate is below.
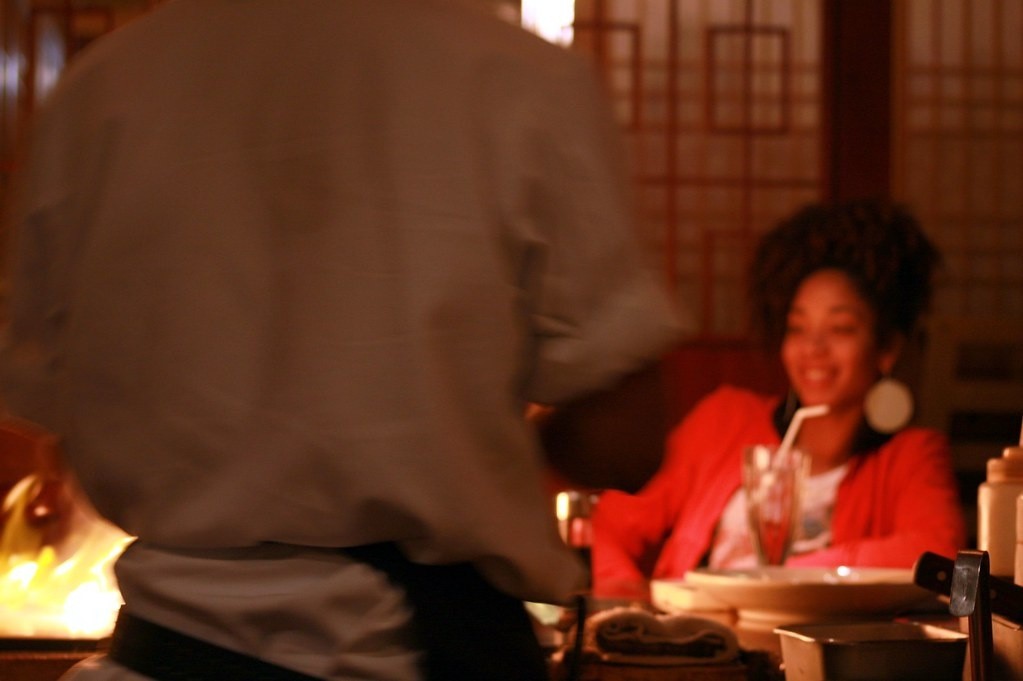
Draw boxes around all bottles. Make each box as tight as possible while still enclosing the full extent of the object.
[978,448,1022,576]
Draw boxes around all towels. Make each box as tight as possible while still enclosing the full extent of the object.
[568,609,738,666]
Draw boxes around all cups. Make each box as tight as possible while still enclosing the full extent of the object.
[745,445,811,568]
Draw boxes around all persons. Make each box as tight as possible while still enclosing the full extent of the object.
[0,0,689,681]
[587,188,966,631]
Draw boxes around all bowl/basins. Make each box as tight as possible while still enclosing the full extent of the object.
[650,579,701,616]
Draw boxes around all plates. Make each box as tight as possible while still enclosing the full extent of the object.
[0,529,134,641]
[684,567,918,627]
